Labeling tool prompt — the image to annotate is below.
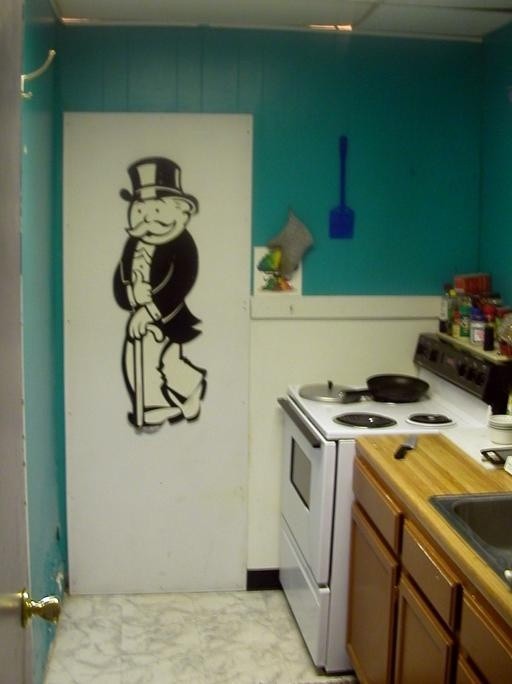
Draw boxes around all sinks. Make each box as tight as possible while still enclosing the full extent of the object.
[426,490,512,592]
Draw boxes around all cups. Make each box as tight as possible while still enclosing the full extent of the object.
[488,414,512,445]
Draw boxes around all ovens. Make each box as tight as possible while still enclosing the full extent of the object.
[275,392,354,676]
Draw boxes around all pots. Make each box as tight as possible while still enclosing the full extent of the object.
[337,374,429,400]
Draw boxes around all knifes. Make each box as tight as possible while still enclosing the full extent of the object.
[392,432,417,462]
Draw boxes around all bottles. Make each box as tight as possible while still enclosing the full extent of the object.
[437,284,512,358]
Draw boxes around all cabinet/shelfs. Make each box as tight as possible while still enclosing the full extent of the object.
[344,432,512,684]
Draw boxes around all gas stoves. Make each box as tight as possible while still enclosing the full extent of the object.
[288,380,456,441]
